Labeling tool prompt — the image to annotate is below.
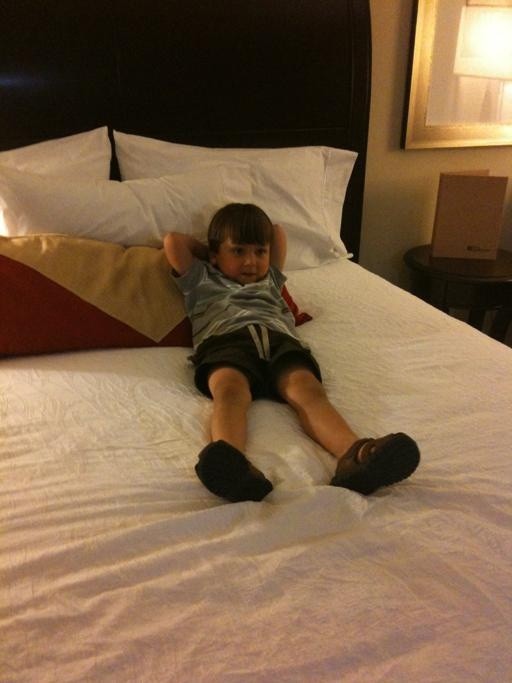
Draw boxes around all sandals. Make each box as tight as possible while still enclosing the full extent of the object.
[328,433,420,495]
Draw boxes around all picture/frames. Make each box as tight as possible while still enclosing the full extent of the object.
[400,1,511,151]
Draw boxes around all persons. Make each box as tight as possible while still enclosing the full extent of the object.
[163,202,421,503]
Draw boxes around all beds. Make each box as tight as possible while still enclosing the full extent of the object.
[0,1,512,682]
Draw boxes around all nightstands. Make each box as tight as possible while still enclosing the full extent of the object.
[403,244,511,347]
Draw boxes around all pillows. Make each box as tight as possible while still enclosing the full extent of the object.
[113,131,359,270]
[1,160,252,248]
[0,126,113,181]
[1,233,315,357]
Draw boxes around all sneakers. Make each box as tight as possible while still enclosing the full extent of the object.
[194,440,273,502]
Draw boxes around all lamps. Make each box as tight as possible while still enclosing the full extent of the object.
[454,3,511,82]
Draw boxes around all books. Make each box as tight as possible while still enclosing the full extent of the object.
[431,166,508,260]
